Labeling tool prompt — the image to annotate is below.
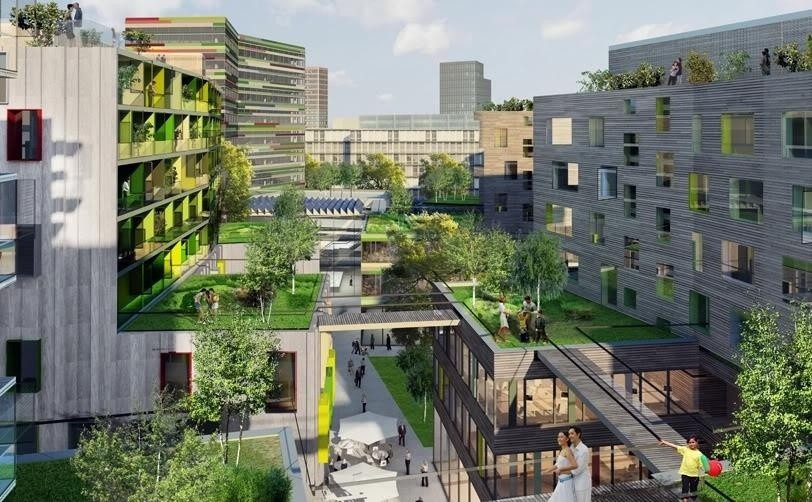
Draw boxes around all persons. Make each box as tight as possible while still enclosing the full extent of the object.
[420,459,428,487]
[122,177,130,203]
[658,433,710,502]
[404,450,411,475]
[73,1,83,27]
[515,296,548,344]
[148,81,156,107]
[759,48,771,77]
[540,431,578,502]
[361,392,367,412]
[347,334,376,388]
[667,56,682,85]
[398,421,406,447]
[560,426,592,502]
[193,287,220,324]
[495,295,511,343]
[63,3,73,29]
[386,333,392,351]
[174,129,181,148]
[156,54,166,63]
